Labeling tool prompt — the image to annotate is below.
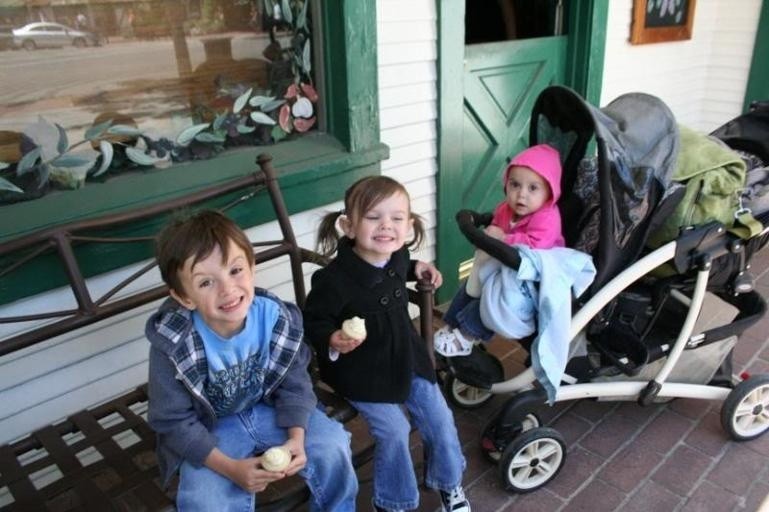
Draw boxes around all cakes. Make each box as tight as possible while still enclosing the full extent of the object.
[262,446,290,472]
[341,316,366,341]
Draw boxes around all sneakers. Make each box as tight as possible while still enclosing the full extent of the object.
[433,484,472,512]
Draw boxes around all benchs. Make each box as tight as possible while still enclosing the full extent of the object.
[0,153,437,512]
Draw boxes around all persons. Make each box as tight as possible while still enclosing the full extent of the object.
[299,174,475,512]
[427,141,566,358]
[144,204,359,510]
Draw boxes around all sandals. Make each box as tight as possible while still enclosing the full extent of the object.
[433,323,473,358]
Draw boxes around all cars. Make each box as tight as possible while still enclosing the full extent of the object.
[9,21,91,49]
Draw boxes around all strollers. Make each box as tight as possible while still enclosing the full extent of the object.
[436,85,769,494]
